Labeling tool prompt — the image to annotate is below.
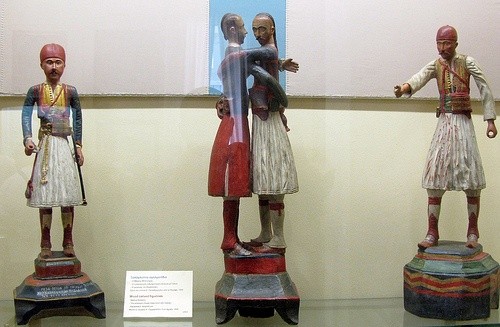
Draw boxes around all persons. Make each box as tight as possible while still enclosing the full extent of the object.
[21,43,87,260]
[394,26,497,249]
[208,12,301,256]
